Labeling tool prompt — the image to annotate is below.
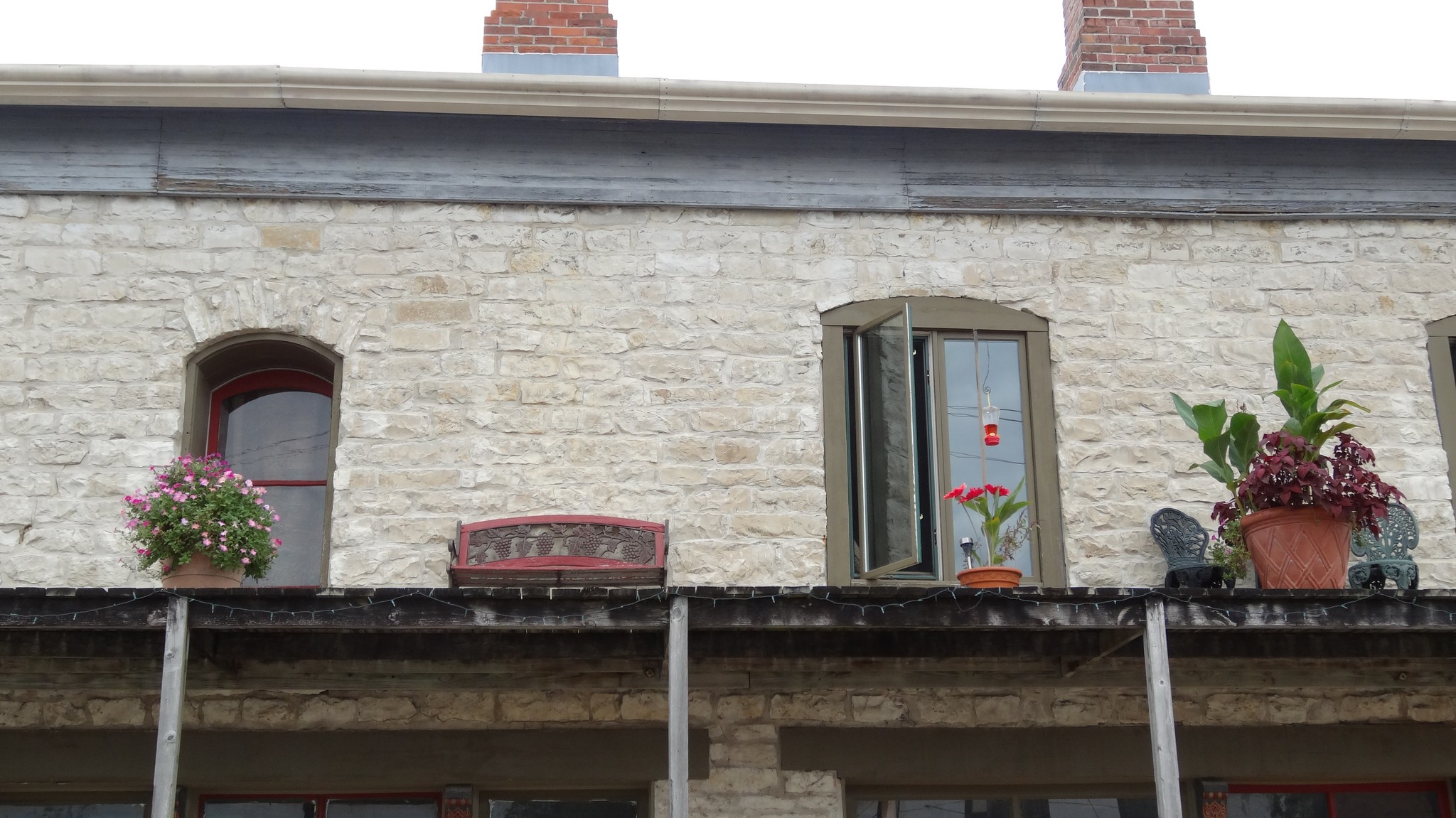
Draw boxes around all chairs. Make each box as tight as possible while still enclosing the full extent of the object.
[1149,508,1236,588]
[1348,503,1419,589]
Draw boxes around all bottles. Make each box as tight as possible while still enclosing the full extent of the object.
[981,407,1000,446]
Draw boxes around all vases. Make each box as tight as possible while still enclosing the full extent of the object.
[956,567,1022,588]
[161,550,244,588]
[1238,505,1353,589]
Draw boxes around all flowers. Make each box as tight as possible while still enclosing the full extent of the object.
[942,477,1041,566]
[113,452,283,582]
[1168,318,1407,576]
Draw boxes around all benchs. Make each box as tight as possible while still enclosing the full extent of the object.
[447,515,670,587]
